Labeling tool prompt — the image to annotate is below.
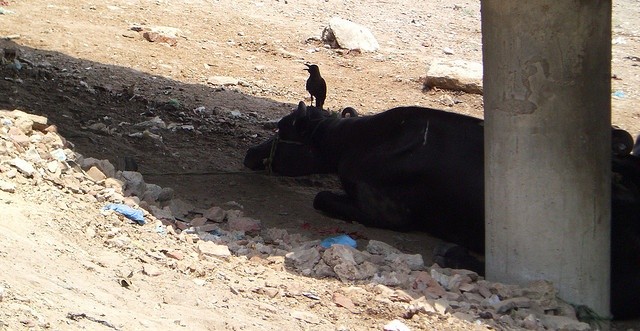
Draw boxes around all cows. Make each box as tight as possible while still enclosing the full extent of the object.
[245,101,639,269]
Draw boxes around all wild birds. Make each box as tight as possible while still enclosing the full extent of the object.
[302,64,327,109]
